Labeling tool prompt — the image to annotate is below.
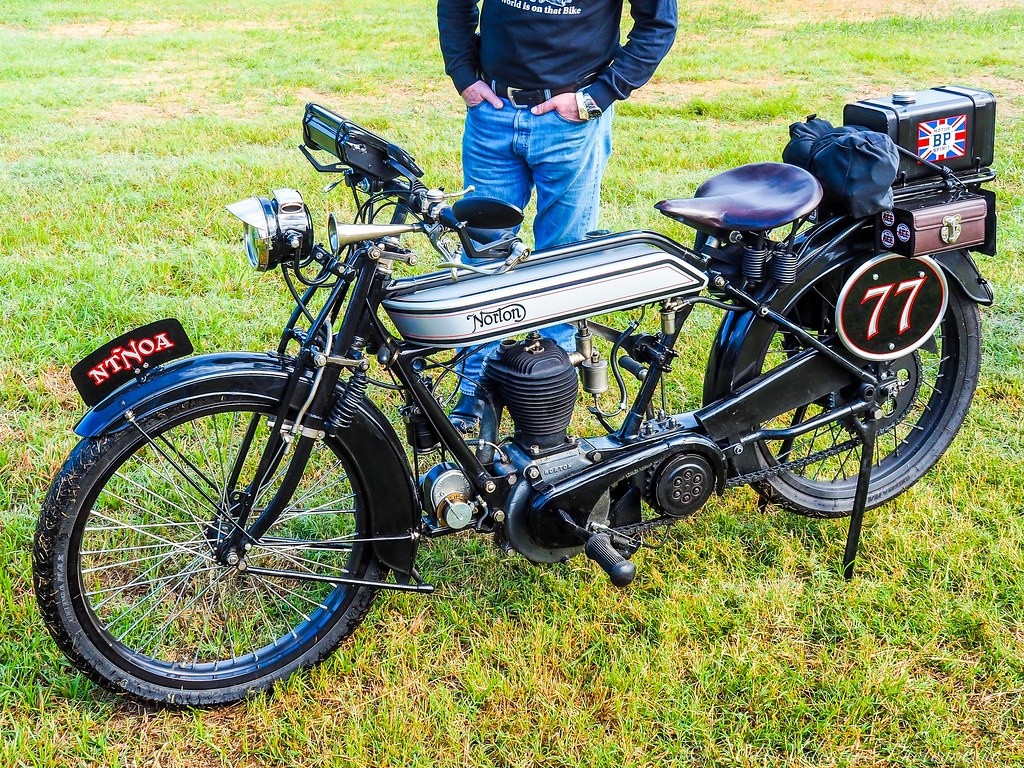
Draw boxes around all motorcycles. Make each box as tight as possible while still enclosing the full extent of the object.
[32,78,1000,712]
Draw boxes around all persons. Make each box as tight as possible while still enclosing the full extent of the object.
[436,1,677,431]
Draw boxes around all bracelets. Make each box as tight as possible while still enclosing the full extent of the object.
[576,91,590,121]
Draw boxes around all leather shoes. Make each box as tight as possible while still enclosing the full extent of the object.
[448,386,485,433]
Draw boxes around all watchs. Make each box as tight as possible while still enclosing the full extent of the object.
[584,93,602,121]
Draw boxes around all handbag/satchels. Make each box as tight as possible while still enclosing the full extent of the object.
[782,115,901,220]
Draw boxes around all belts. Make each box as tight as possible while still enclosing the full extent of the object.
[478,74,594,109]
